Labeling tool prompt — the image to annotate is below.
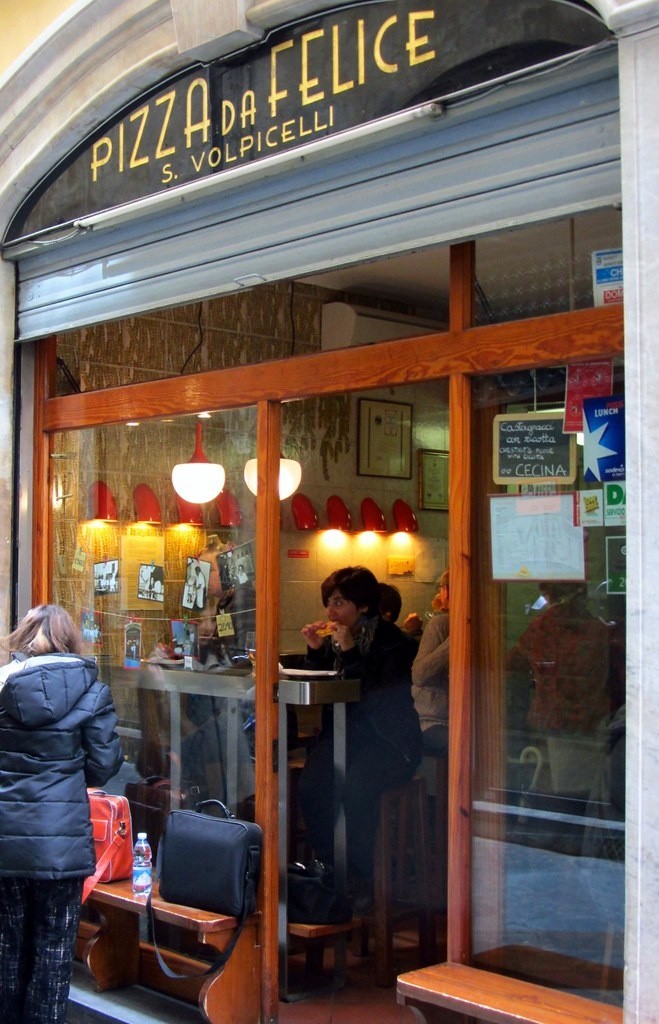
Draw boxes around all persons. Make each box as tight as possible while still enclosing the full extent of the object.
[299,565,424,981]
[138,580,254,818]
[0,602,124,1024]
[192,566,207,610]
[235,565,248,583]
[411,607,449,806]
[147,571,156,592]
[373,577,406,622]
[146,632,182,678]
[513,567,614,741]
[430,567,455,616]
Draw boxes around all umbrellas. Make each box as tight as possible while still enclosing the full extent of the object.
[501,747,549,846]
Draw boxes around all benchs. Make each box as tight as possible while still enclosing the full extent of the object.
[83,875,262,1024]
[396,961,624,1024]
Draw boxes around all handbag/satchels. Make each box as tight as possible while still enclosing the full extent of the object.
[287,860,352,924]
[146,799,263,981]
[81,787,134,883]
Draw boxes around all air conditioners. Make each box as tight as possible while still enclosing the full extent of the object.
[321,301,450,351]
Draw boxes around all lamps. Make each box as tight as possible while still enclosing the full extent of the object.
[134,483,162,524]
[213,487,244,528]
[393,498,419,533]
[361,497,387,533]
[72,103,445,235]
[88,480,118,523]
[325,494,353,531]
[243,452,302,501]
[172,422,226,504]
[175,493,204,526]
[290,493,320,531]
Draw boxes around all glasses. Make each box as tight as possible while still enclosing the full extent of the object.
[436,585,444,593]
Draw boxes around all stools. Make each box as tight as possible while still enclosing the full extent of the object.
[421,747,449,874]
[350,773,436,989]
[287,914,366,986]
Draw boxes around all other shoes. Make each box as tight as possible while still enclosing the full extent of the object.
[348,862,374,916]
